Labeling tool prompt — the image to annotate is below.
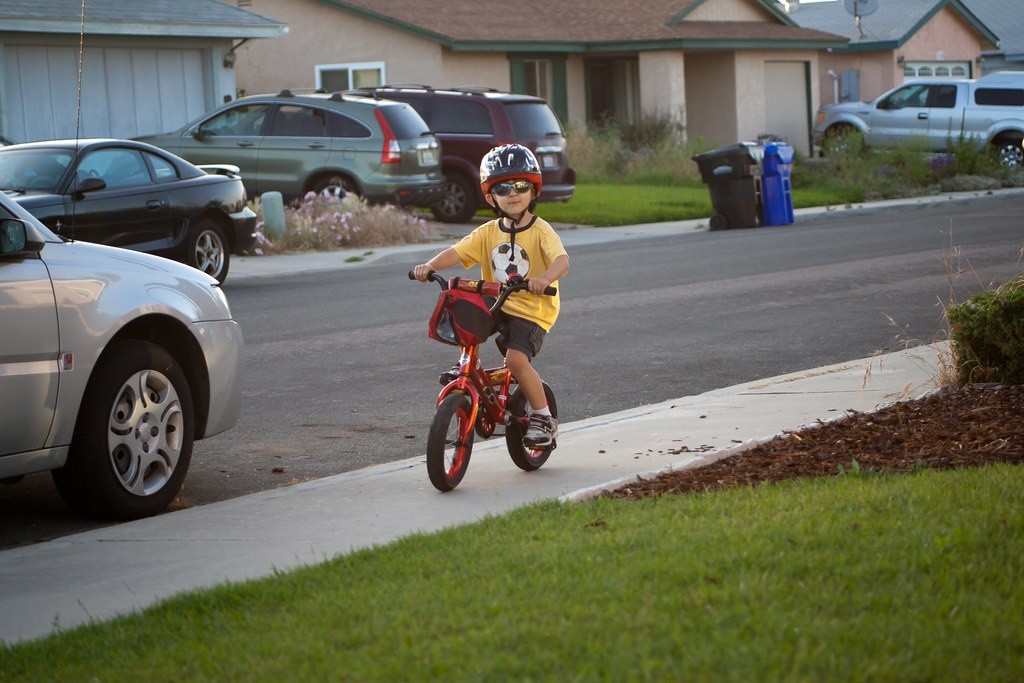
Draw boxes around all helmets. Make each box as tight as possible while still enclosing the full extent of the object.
[480,143,542,198]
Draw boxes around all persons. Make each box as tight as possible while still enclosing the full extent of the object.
[414,144,570,448]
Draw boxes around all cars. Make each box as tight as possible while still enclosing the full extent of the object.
[0,190,244,523]
[0,137,257,288]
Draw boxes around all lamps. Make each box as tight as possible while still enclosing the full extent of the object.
[223,53,236,68]
[897,56,905,64]
[974,57,986,64]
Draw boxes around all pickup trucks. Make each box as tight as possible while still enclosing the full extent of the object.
[811,71,1024,173]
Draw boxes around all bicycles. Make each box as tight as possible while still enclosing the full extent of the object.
[407,268,558,494]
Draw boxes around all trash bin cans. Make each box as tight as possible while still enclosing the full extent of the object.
[690,142,767,231]
[754,142,794,227]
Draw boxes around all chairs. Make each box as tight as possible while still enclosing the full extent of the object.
[99,155,140,188]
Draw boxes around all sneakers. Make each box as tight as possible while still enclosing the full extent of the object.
[439,358,482,396]
[522,413,560,446]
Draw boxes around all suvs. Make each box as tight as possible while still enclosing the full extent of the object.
[289,82,577,226]
[126,87,448,211]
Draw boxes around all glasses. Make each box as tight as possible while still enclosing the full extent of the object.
[491,180,534,196]
